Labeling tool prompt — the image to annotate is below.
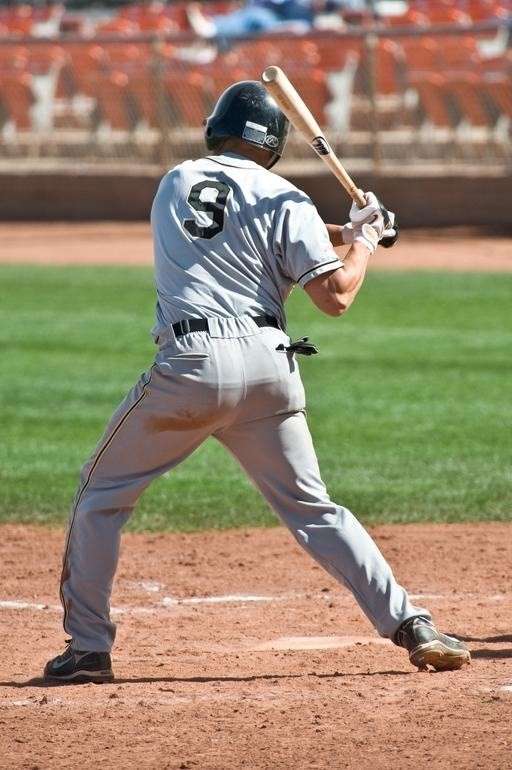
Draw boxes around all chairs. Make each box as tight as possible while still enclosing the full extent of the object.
[0,1,512,160]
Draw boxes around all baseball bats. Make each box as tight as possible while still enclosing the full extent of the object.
[262,66,396,251]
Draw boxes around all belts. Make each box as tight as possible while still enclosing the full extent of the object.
[170,314,281,337]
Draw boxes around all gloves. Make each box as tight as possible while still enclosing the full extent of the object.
[277,336,319,355]
[341,188,397,255]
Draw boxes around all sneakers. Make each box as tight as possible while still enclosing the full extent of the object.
[43,648,115,682]
[395,616,471,670]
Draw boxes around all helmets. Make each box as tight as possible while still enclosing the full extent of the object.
[202,80,290,159]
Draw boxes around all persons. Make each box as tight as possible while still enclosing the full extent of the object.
[314,1,407,131]
[43,80,471,683]
[173,0,314,65]
